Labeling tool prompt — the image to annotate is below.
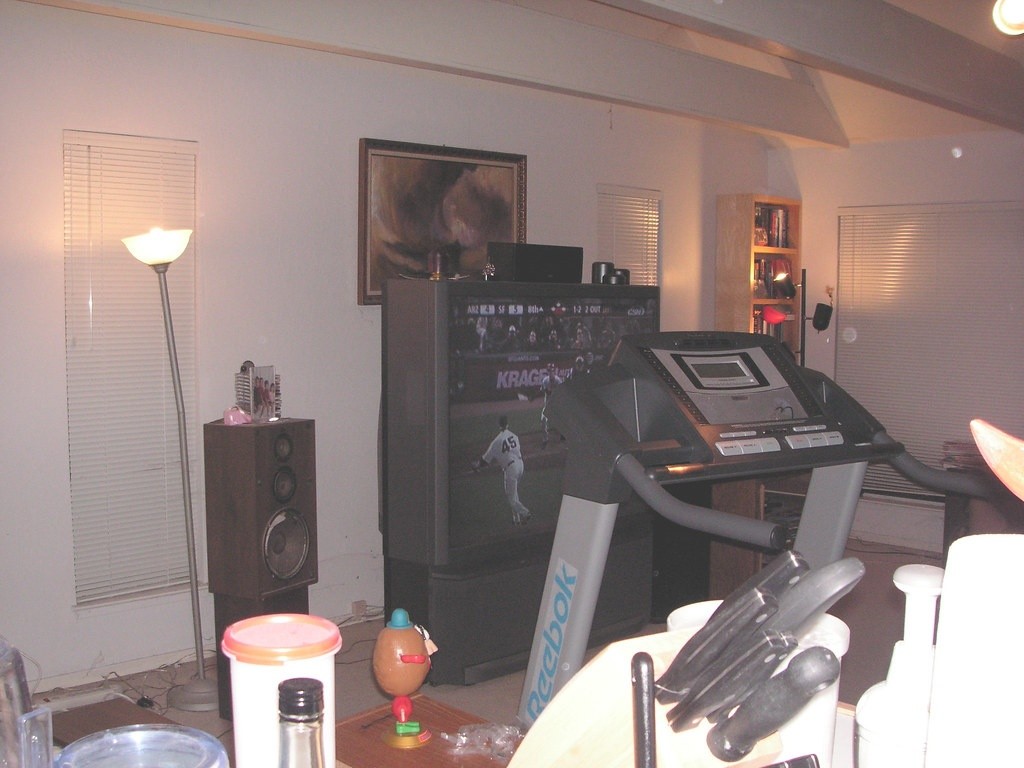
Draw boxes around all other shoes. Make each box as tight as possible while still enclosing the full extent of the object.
[521,512,531,524]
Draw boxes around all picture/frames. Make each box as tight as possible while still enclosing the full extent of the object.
[358,137,527,306]
[249,365,275,421]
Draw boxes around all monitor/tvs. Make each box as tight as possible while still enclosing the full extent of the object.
[380,277,661,579]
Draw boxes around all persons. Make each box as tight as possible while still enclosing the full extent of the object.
[470,415,532,529]
[252,375,276,422]
[538,361,567,451]
[465,316,644,354]
[567,356,592,385]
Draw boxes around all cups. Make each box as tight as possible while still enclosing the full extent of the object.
[667,600,850,768]
[220,613,342,768]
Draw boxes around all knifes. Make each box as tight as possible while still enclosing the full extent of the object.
[631,548,865,768]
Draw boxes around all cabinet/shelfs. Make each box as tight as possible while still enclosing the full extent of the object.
[715,193,806,366]
[486,241,583,284]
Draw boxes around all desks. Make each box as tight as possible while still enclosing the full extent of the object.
[942,467,1024,569]
[336,689,526,768]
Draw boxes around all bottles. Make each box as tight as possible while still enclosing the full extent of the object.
[0,643,36,768]
[278,678,323,768]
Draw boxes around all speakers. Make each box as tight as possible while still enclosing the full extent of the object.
[213,587,309,720]
[203,418,319,600]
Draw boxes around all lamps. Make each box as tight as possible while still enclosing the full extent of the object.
[773,268,832,366]
[123,227,247,711]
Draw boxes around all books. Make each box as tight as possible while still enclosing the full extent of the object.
[754,204,795,351]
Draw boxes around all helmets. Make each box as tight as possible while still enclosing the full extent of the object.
[576,357,585,364]
[547,363,556,372]
